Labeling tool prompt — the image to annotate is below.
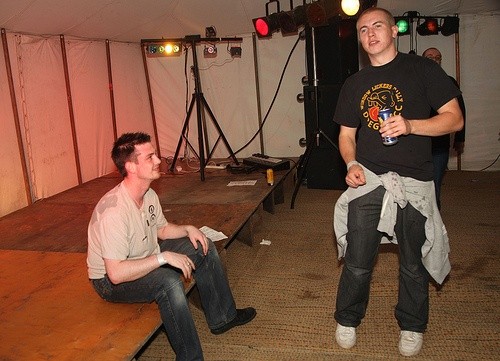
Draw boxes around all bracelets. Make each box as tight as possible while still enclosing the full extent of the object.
[347,161,359,171]
[403,119,411,135]
[156,253,167,266]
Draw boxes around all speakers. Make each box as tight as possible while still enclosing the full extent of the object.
[303,84,349,191]
[305,17,360,86]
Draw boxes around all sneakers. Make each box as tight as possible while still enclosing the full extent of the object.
[398,330,422,357]
[335,324,357,349]
[211,307,256,335]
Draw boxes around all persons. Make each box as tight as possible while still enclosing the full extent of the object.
[334,7,464,356]
[87,132,257,361]
[421,48,466,211]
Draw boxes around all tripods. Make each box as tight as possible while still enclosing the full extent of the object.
[290,26,340,210]
[170,39,240,181]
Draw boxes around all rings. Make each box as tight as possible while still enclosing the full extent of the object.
[187,262,191,265]
